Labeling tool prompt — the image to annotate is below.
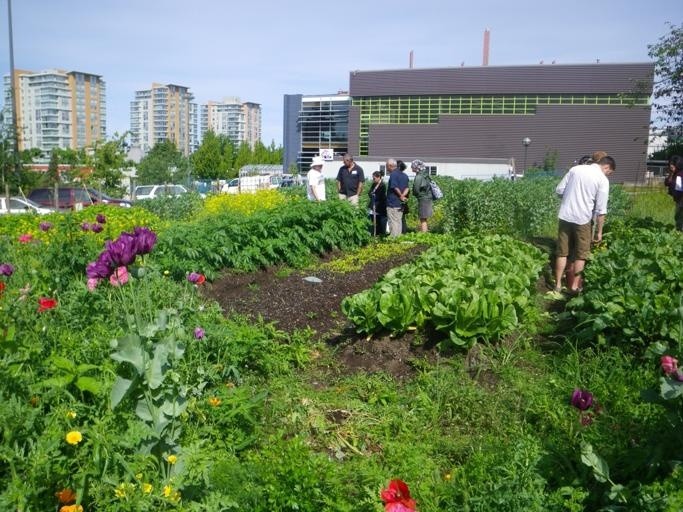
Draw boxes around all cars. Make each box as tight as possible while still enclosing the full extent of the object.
[0,196,57,216]
[27,186,133,209]
[133,182,206,201]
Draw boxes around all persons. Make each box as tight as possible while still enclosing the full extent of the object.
[554,157,617,291]
[664,155,683,231]
[306,156,327,202]
[397,160,410,234]
[569,150,609,289]
[386,158,410,237]
[578,155,593,164]
[411,159,433,231]
[368,169,387,236]
[335,153,366,208]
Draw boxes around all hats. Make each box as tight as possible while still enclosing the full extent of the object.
[310,156,325,167]
[411,159,426,171]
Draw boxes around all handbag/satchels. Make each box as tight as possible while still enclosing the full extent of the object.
[429,179,443,199]
[402,204,409,214]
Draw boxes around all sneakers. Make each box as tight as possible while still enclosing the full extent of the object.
[553,287,581,295]
